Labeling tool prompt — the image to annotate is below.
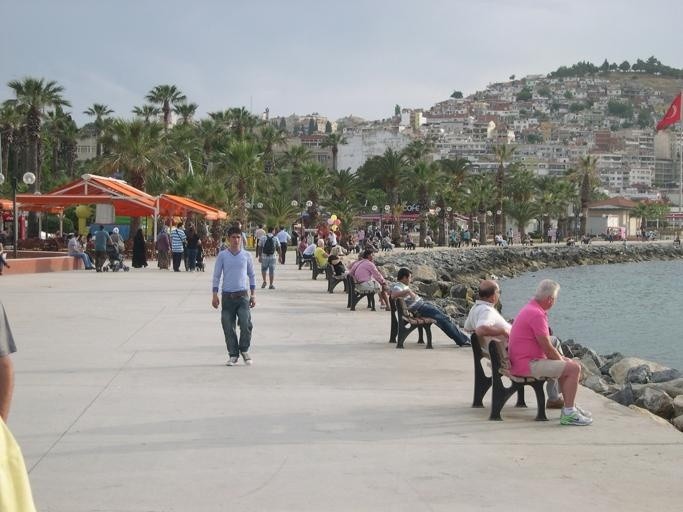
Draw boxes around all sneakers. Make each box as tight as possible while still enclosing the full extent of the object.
[262,282,266,288]
[380,304,391,311]
[240,351,252,365]
[560,406,593,426]
[460,341,473,347]
[226,357,239,367]
[546,399,564,409]
[270,285,274,288]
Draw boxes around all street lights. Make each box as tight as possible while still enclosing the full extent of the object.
[569,208,583,242]
[485,209,502,246]
[244,201,263,247]
[370,204,390,244]
[290,199,313,242]
[0,170,37,258]
[434,206,452,247]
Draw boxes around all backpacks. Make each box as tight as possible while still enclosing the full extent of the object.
[263,234,275,254]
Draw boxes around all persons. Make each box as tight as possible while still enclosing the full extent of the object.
[606,229,614,243]
[567,234,585,246]
[505,279,595,427]
[132,229,148,268]
[451,229,478,246]
[462,279,567,409]
[68,225,124,272]
[389,267,473,349]
[548,227,561,243]
[523,234,530,244]
[298,227,394,275]
[348,250,392,311]
[426,233,432,245]
[235,221,299,290]
[156,222,201,272]
[0,297,16,427]
[0,242,10,276]
[211,224,256,368]
[495,228,513,246]
[405,234,414,246]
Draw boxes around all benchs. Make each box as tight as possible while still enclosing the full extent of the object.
[296,252,376,311]
[470,333,549,421]
[346,240,478,254]
[388,296,436,348]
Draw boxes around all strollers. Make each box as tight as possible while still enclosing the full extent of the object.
[102,242,130,274]
[194,244,206,272]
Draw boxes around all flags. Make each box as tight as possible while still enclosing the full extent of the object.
[655,93,682,133]
